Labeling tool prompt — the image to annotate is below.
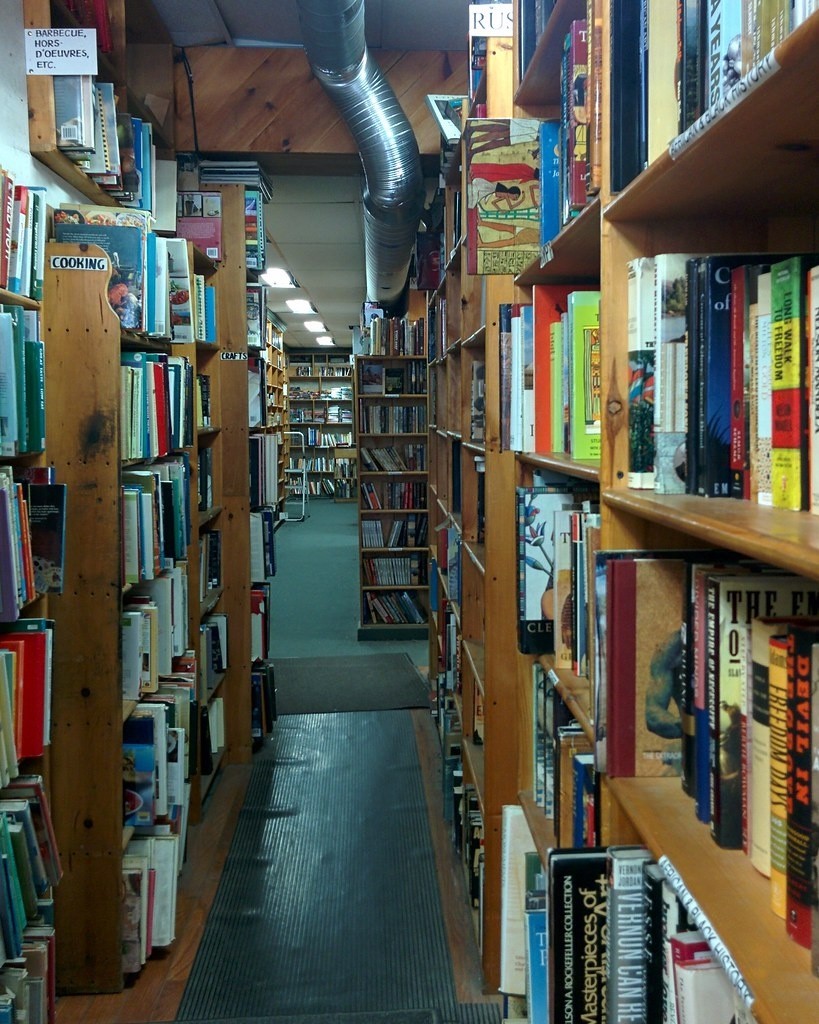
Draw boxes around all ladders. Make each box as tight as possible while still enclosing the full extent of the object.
[284,431,311,522]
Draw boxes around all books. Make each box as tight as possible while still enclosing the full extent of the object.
[0,0,287,1024]
[359,317,428,624]
[428,0,819,1024]
[287,365,357,499]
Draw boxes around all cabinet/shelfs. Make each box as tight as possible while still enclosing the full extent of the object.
[166,240,236,830]
[259,313,358,505]
[35,238,198,991]
[0,274,67,1024]
[423,1,818,1023]
[350,354,429,647]
[22,0,176,227]
[179,177,283,772]
